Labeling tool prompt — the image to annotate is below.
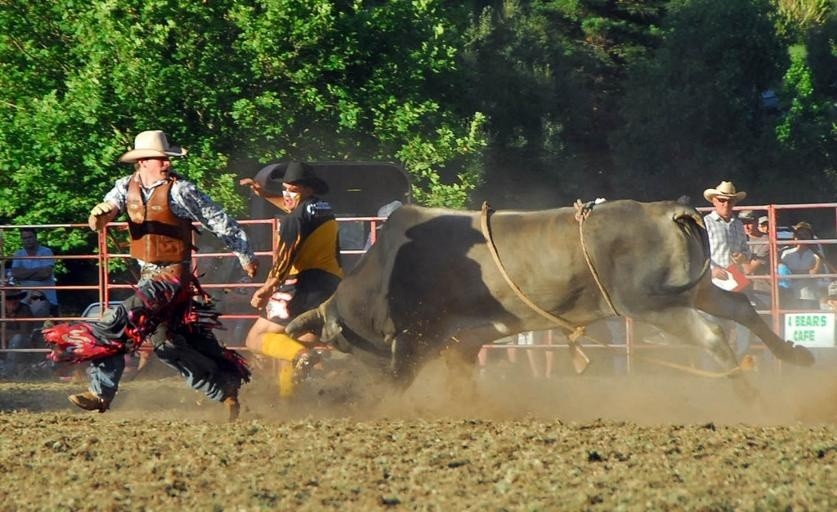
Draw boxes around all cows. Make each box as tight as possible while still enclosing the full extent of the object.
[284,201,815,409]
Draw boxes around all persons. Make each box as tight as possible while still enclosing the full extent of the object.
[734,208,836,315]
[196,244,264,347]
[0,227,61,383]
[688,180,752,373]
[476,317,639,397]
[238,159,347,406]
[63,127,260,424]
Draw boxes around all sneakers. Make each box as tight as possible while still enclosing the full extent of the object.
[68,390,110,413]
[290,347,320,385]
[224,383,241,421]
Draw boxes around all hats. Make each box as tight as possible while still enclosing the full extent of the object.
[758,216,769,224]
[0,284,28,300]
[737,210,756,221]
[118,130,188,163]
[269,161,328,195]
[703,180,746,203]
[792,222,814,232]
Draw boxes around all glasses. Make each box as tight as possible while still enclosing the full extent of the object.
[718,198,735,202]
[31,295,46,301]
[280,185,299,192]
